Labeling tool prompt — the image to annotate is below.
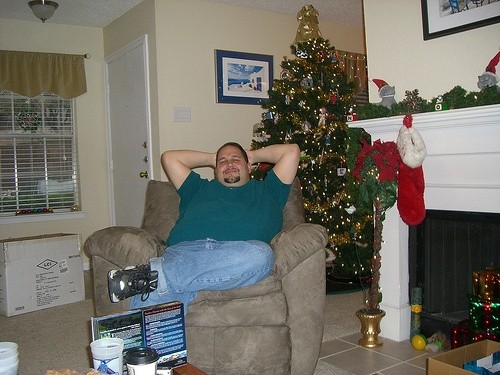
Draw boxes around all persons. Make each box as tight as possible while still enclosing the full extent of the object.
[106,142,301,318]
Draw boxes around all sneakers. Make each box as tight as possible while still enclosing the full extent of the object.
[107,264,158,304]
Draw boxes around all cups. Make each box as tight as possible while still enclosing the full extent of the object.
[0,341,20,375]
[124,347,159,375]
[89,338,125,375]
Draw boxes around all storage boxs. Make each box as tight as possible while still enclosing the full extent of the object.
[427,339,500,375]
[0,234,84,316]
[91,301,188,370]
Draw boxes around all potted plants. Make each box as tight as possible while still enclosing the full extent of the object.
[347,162,399,347]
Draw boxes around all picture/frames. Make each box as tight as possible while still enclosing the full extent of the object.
[422,0,500,45]
[215,50,275,104]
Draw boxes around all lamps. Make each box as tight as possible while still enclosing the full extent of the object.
[27,1,63,27]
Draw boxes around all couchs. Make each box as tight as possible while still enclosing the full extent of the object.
[86,181,328,375]
